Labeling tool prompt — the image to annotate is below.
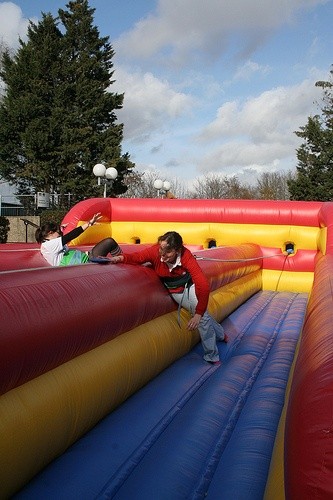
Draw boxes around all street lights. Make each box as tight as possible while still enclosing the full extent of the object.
[153,179,171,198]
[93,163,118,199]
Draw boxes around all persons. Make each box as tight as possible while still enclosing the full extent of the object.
[36,213,125,265]
[97,232,227,365]
